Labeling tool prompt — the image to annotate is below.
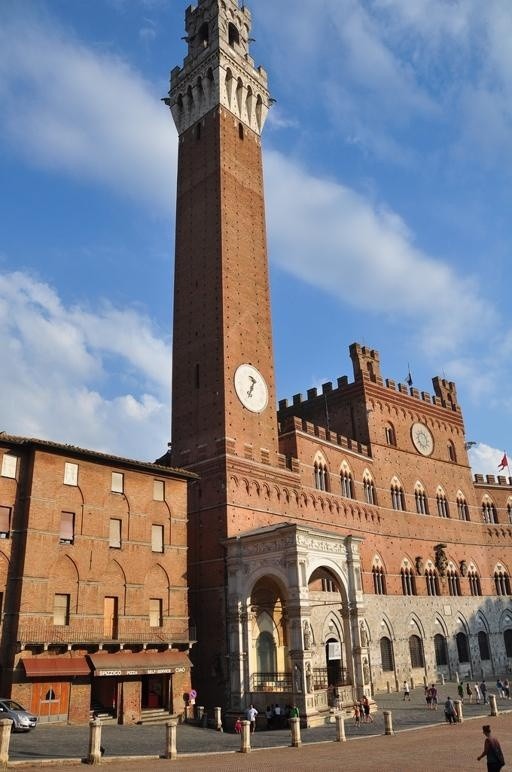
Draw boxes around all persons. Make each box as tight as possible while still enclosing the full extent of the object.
[352,705,360,727]
[400,680,410,701]
[234,718,242,734]
[243,702,292,730]
[466,683,472,704]
[502,677,511,700]
[445,696,458,725]
[443,703,452,722]
[424,686,433,710]
[246,703,259,734]
[474,682,481,704]
[476,724,505,772]
[495,677,505,699]
[288,703,299,736]
[480,680,489,705]
[457,681,464,704]
[360,695,374,723]
[328,683,334,688]
[354,697,366,723]
[426,683,438,712]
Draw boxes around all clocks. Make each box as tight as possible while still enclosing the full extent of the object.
[234,363,269,413]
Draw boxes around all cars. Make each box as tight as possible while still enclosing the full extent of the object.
[0,698,38,733]
